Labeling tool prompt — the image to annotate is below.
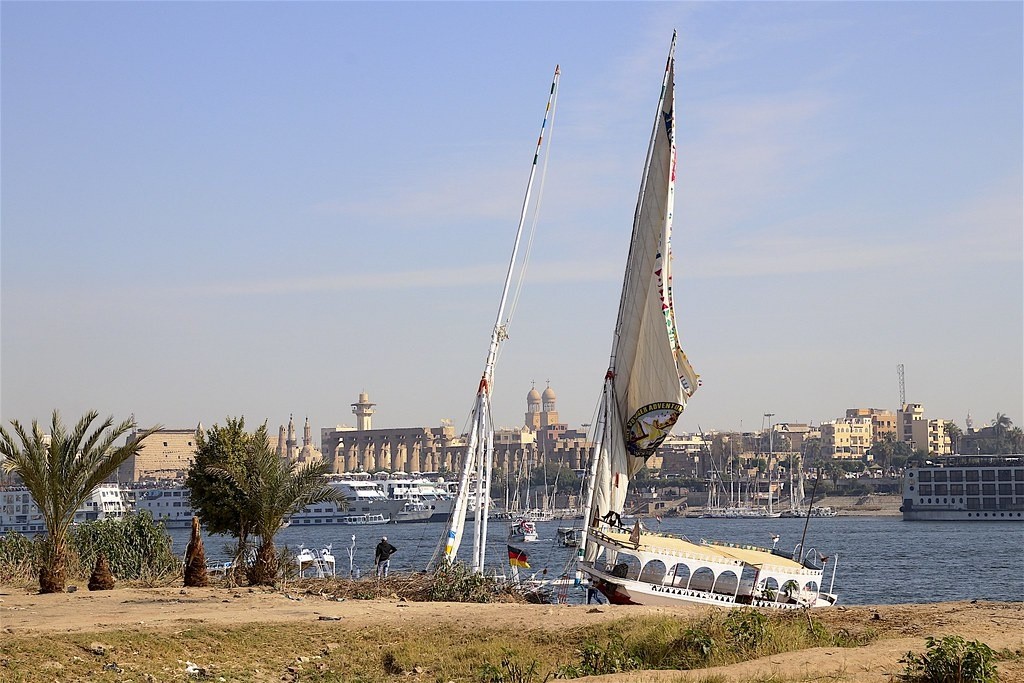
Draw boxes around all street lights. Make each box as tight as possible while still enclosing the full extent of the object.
[764,413,775,513]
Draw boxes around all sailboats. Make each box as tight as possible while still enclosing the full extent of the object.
[580,28,839,611]
[686,420,837,518]
[505,435,635,523]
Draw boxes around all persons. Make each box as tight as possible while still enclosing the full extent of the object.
[376,537,397,578]
[588,586,602,604]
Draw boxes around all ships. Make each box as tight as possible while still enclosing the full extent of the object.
[367,471,491,523]
[0,480,136,535]
[136,465,408,525]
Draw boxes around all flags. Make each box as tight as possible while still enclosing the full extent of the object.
[508,545,530,568]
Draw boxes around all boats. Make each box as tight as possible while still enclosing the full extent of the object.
[559,525,646,548]
[509,518,539,542]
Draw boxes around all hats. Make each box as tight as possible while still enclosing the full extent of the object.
[382,537,387,540]
